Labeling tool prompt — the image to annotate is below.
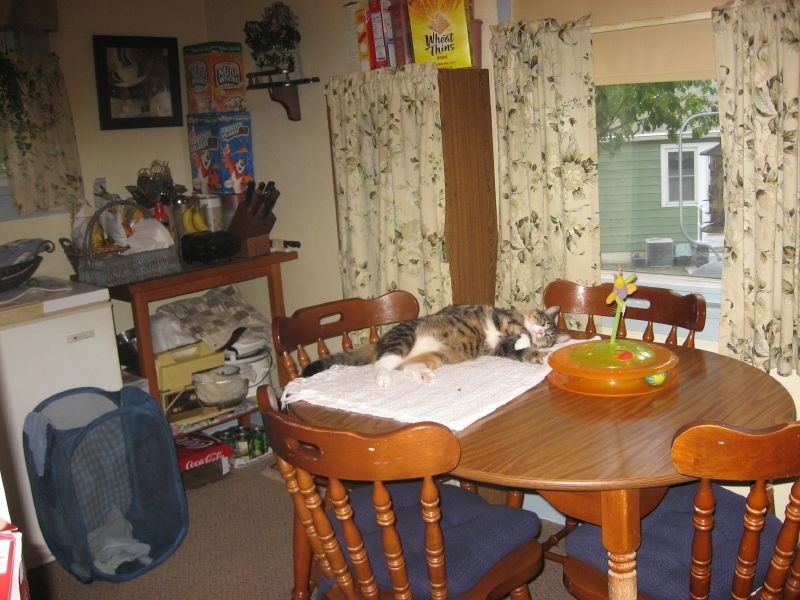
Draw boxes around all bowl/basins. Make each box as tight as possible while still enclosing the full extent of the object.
[193,365,249,408]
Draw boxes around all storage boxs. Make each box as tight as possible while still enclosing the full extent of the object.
[187,110,254,195]
[182,40,249,114]
[123,327,224,419]
[340,0,475,70]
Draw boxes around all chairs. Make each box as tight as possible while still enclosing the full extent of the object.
[257,280,800,600]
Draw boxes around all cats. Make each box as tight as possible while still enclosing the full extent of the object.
[298,305,574,389]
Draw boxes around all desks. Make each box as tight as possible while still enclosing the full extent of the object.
[291,331,796,600]
[63,248,300,445]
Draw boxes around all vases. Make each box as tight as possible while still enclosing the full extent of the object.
[257,48,299,81]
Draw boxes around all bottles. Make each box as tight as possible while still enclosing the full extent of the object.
[154,200,171,235]
[173,195,191,239]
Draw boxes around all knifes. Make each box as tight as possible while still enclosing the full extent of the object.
[243,180,279,220]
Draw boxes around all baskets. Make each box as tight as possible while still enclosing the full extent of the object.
[77,199,184,287]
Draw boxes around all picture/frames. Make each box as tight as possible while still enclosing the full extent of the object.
[93,34,184,130]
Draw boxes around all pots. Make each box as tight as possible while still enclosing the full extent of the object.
[181,231,239,263]
[0,254,44,295]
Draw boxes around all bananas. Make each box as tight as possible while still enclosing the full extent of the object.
[182,204,197,233]
[192,204,209,231]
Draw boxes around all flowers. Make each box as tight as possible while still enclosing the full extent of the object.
[244,2,302,69]
